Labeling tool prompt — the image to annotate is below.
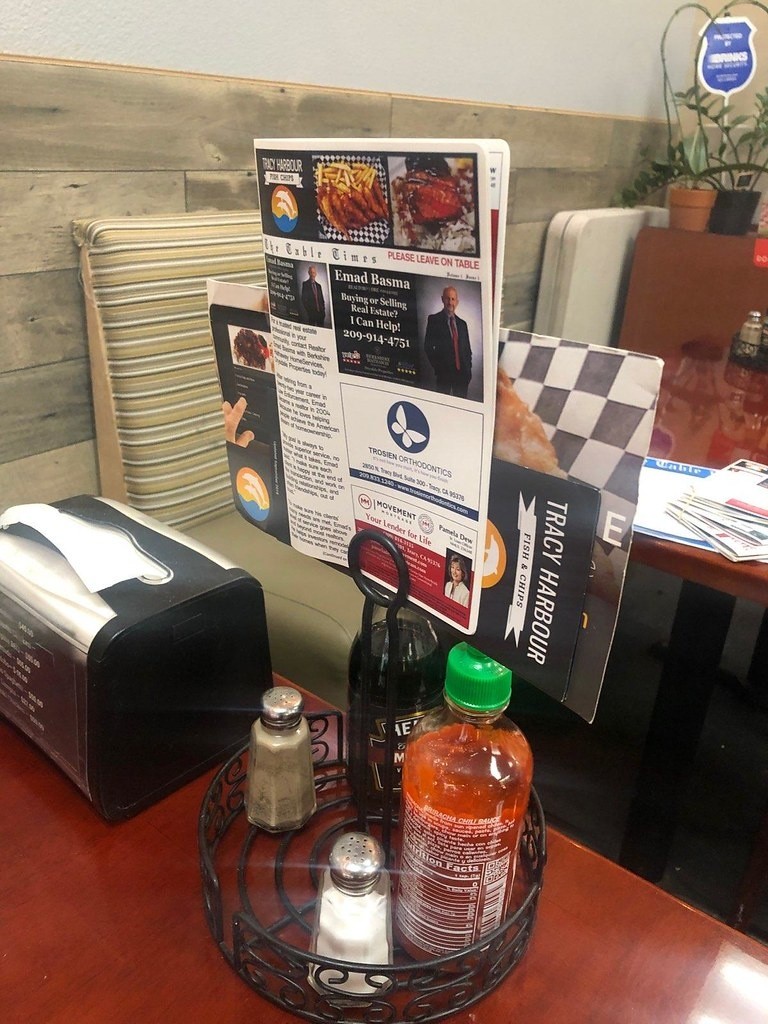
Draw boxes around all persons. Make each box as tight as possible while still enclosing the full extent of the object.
[444,554,469,609]
[423,286,473,397]
[302,266,326,327]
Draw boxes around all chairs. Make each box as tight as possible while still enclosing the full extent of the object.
[72,212,449,714]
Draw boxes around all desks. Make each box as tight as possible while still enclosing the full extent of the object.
[592,226,768,885]
[0,669,768,1024]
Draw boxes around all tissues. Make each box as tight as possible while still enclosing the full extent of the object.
[0,492,275,828]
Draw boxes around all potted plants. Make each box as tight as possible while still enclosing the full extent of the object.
[613,0,768,236]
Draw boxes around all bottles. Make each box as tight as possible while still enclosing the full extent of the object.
[393,642,533,972]
[245,686,318,834]
[346,600,446,818]
[308,830,394,1006]
[736,310,763,358]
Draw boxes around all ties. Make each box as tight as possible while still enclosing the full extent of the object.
[449,316,461,373]
[312,282,320,312]
